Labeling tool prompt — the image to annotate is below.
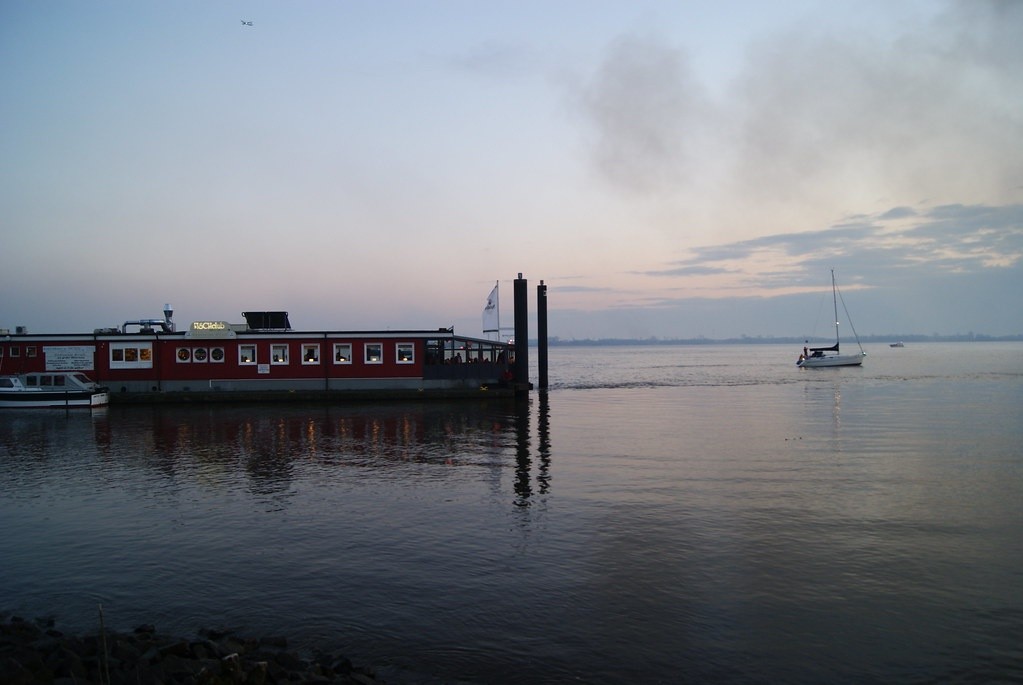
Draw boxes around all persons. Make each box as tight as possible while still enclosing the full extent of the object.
[799,354,803,362]
[336,352,350,361]
[455,353,462,363]
[274,354,279,362]
[54,376,60,385]
[40,376,50,385]
[496,350,505,364]
[304,353,318,362]
[469,357,491,362]
[27,380,34,385]
[241,356,247,362]
[804,346,808,356]
[398,349,405,361]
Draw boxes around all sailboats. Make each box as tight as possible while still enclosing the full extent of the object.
[794,268,867,369]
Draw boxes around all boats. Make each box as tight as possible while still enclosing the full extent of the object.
[889,340,905,348]
[1,371,108,408]
[0,302,533,401]
